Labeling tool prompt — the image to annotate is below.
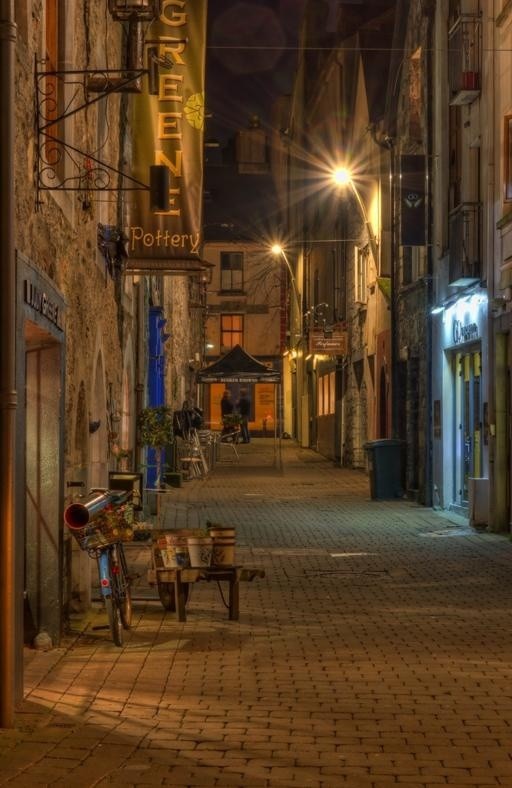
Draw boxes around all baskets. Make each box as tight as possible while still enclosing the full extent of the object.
[106,504,135,541]
[69,517,119,550]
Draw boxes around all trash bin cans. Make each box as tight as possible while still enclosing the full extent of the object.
[363,438,407,501]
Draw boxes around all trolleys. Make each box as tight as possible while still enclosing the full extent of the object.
[146,564,263,622]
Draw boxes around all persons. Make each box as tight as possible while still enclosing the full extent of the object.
[219,389,235,442]
[235,386,251,444]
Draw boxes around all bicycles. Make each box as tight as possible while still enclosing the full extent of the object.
[65,487,142,643]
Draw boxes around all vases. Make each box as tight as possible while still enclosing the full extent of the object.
[158,528,236,569]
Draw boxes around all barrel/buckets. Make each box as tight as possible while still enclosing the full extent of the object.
[157,525,237,566]
